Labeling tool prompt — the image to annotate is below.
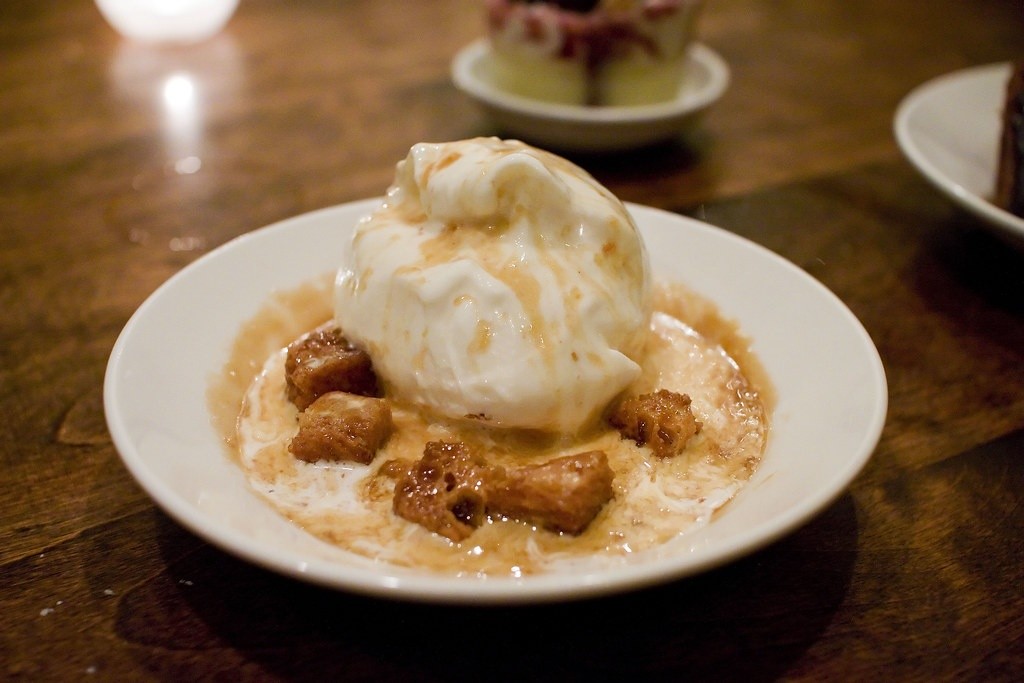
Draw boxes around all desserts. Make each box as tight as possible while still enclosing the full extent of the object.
[479,0,704,108]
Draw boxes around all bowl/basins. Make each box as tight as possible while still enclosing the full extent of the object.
[894,62,1024,247]
[101,197,890,603]
[450,41,730,148]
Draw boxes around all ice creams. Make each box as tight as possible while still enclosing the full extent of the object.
[238,133,769,578]
